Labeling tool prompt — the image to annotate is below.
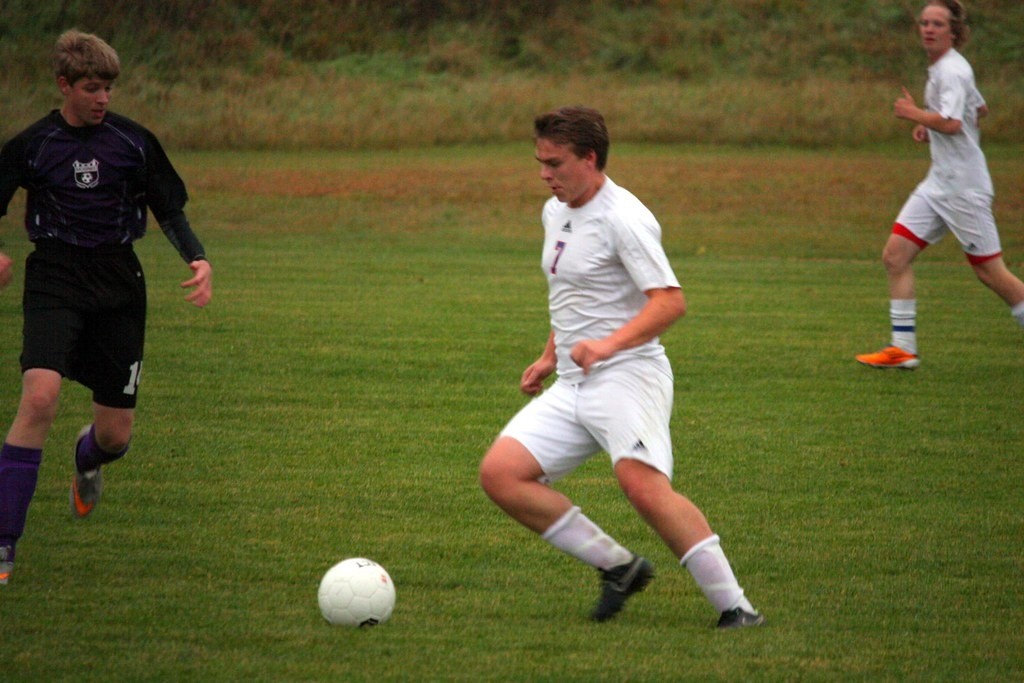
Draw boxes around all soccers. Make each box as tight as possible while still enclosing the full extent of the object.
[316,556,397,628]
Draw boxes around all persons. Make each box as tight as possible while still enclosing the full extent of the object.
[0,33,216,588]
[851,0,1023,369]
[476,103,765,631]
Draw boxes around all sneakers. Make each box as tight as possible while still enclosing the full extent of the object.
[71,426,103,519]
[718,607,768,629]
[1,547,16,586]
[593,555,656,619]
[858,345,920,369]
